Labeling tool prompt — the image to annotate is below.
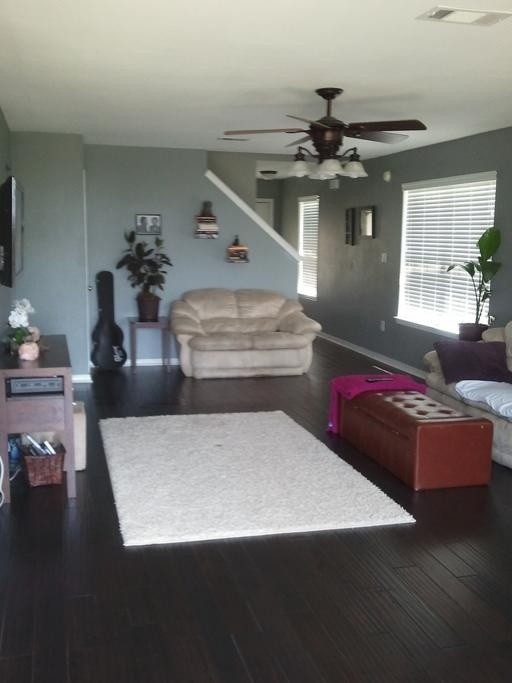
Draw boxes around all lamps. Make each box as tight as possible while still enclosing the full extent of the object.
[287,149,369,182]
[259,168,276,182]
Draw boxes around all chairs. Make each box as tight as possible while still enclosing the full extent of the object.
[169,285,323,380]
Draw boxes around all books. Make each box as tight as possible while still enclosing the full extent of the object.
[194,215,219,235]
[19,433,57,456]
[227,245,248,259]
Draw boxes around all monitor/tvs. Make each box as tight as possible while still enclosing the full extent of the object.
[0,176,24,288]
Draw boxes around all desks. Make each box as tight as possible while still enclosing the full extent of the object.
[1,336,76,502]
[129,314,171,374]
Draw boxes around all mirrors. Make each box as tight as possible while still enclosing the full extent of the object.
[356,206,375,239]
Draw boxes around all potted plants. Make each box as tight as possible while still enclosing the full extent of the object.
[447,226,502,340]
[117,233,173,318]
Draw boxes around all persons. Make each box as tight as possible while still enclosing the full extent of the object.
[149,217,160,233]
[137,217,147,233]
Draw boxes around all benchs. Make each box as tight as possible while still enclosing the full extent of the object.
[329,370,496,493]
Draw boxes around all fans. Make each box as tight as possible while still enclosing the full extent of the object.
[223,86,428,151]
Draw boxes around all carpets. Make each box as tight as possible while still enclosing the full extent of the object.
[98,409,417,546]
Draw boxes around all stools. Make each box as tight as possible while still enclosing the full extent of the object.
[20,397,86,472]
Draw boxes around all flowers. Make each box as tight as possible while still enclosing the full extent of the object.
[7,296,36,329]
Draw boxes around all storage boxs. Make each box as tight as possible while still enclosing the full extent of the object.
[22,439,66,486]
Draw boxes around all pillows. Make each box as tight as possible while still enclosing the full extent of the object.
[433,341,512,386]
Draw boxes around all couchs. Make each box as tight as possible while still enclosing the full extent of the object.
[424,320,512,468]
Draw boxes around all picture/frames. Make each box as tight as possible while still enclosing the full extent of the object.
[135,212,162,236]
